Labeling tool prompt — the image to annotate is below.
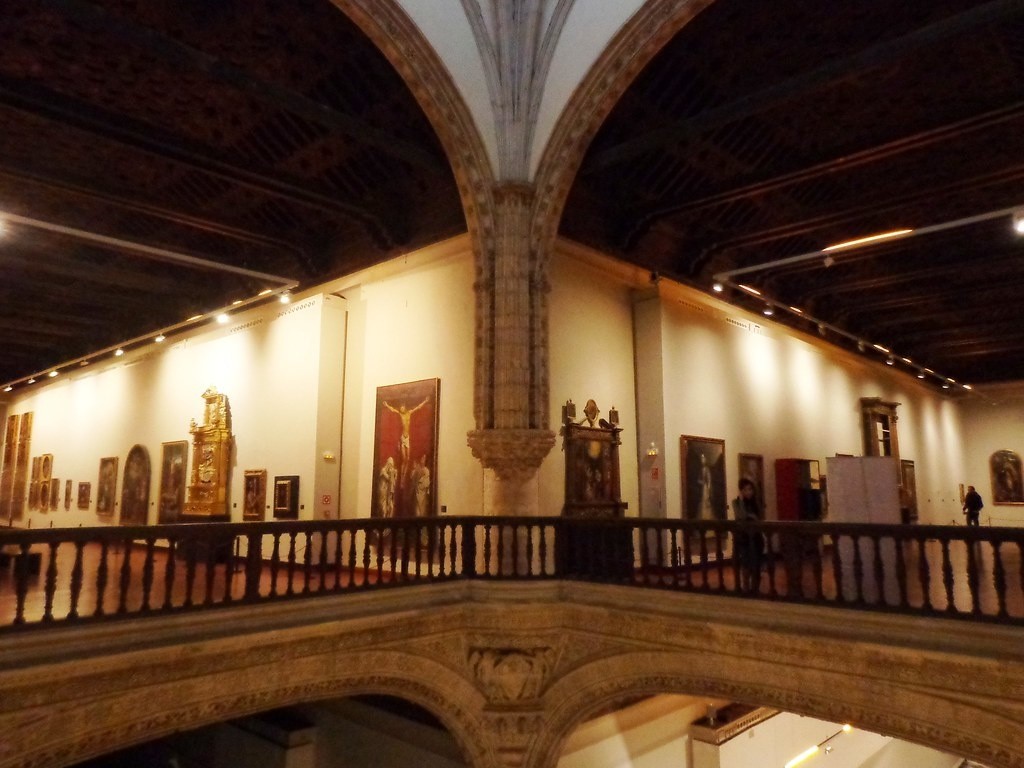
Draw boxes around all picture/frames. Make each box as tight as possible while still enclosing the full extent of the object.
[738,453,765,503]
[0,412,31,520]
[64,480,72,508]
[156,441,187,524]
[49,478,59,507]
[96,457,118,515]
[681,434,728,543]
[370,378,440,546]
[243,471,266,521]
[78,481,89,507]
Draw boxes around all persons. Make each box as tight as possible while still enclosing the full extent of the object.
[963,486,983,527]
[798,472,822,598]
[899,487,910,525]
[732,478,761,593]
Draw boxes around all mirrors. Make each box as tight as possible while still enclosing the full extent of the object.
[989,450,1024,505]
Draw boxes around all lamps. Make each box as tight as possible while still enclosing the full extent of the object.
[887,355,896,365]
[763,304,774,318]
[942,381,951,390]
[916,370,925,380]
